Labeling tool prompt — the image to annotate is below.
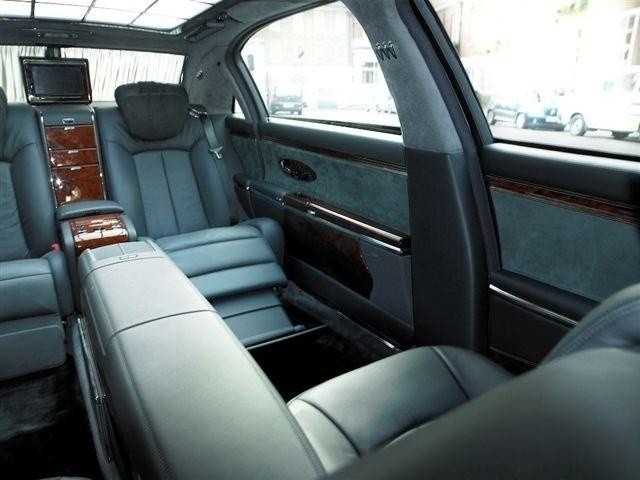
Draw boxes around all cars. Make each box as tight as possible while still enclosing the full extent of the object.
[376,97,398,114]
[485,89,640,138]
[269,94,304,116]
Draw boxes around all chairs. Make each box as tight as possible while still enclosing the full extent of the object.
[0,80,639,479]
